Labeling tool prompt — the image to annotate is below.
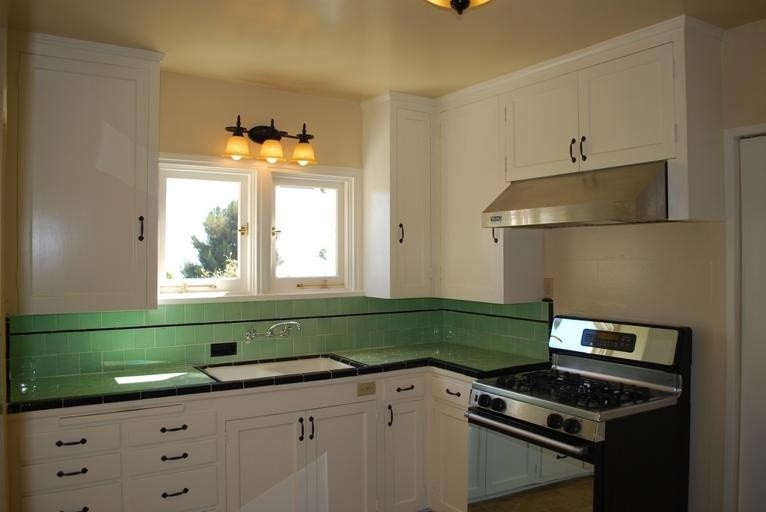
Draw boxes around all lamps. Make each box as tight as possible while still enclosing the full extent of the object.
[425,0,492,16]
[221,113,318,168]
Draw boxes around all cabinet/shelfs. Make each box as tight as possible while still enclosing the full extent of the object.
[466,420,541,502]
[435,83,548,308]
[538,443,595,486]
[9,26,166,317]
[0,398,123,512]
[426,360,484,512]
[382,365,429,512]
[119,387,216,510]
[212,369,385,511]
[354,89,435,303]
[505,14,725,187]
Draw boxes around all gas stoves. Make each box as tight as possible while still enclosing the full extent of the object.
[467,370,678,442]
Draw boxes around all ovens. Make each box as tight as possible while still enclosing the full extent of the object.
[463,412,596,512]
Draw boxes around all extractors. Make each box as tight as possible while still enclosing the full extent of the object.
[482,159,667,229]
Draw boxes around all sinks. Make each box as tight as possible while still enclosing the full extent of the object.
[193,352,370,383]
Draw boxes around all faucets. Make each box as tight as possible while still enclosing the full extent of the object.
[265,321,302,337]
